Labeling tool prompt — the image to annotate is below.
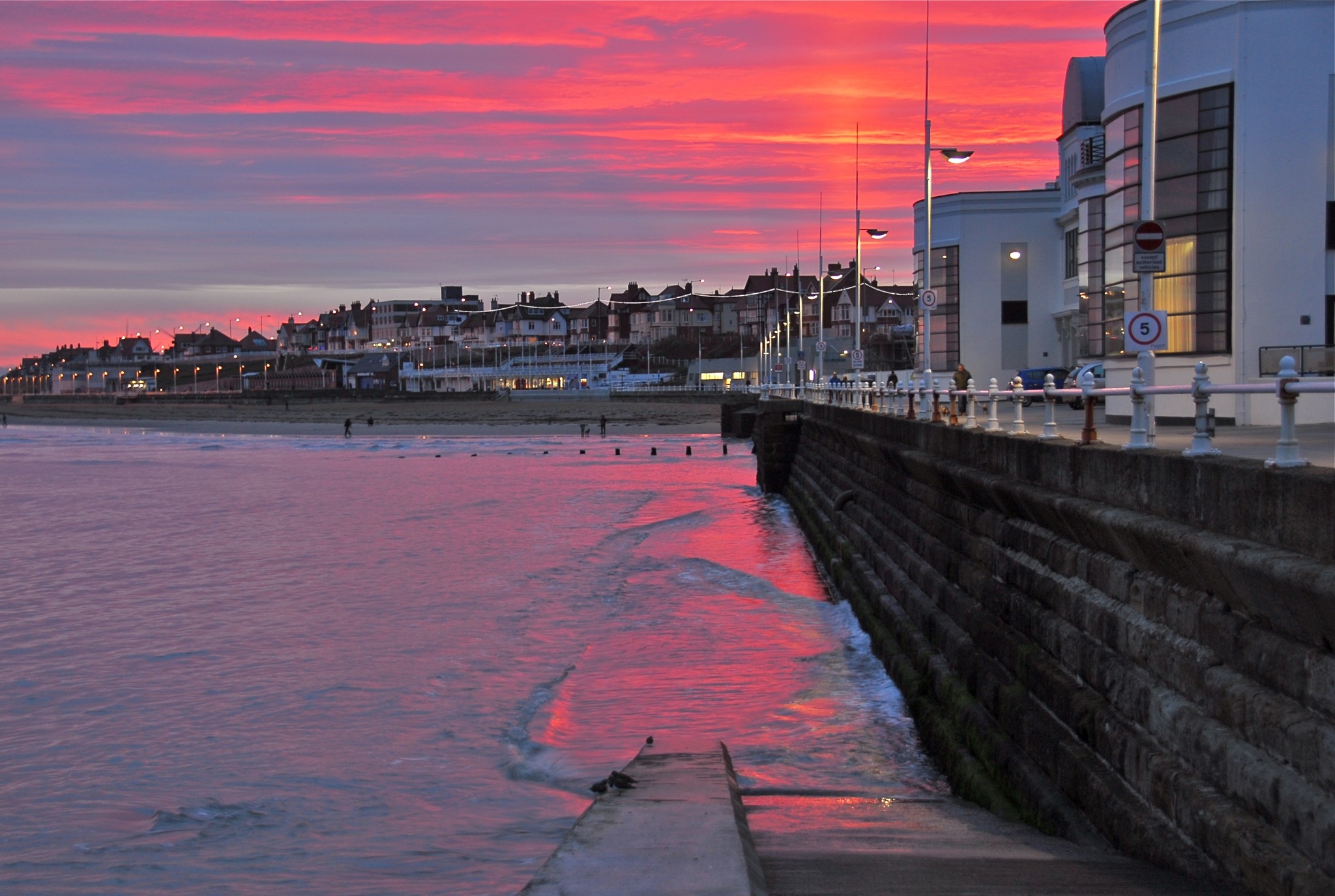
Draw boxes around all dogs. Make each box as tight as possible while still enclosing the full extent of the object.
[939,404,951,416]
[982,404,988,414]
[585,428,590,437]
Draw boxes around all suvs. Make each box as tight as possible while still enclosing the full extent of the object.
[1010,367,1071,408]
[1061,362,1106,410]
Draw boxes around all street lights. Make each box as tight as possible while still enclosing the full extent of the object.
[260,315,271,351]
[548,318,555,366]
[739,319,750,386]
[816,191,844,397]
[597,286,611,344]
[199,322,210,358]
[849,123,891,403]
[784,255,800,384]
[697,320,708,391]
[919,0,975,420]
[773,275,788,383]
[453,296,466,341]
[689,279,706,343]
[758,305,769,385]
[290,312,302,351]
[149,329,160,351]
[2,333,271,394]
[173,326,184,358]
[229,318,240,353]
[647,327,656,390]
[767,292,781,384]
[763,295,770,384]
[796,230,819,399]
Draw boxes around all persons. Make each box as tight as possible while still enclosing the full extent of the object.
[2,414,7,425]
[841,376,848,386]
[599,415,607,434]
[745,379,751,390]
[712,382,717,390]
[952,364,972,416]
[367,416,373,426]
[580,423,587,434]
[772,379,777,384]
[829,371,841,399]
[344,418,352,435]
[887,371,898,402]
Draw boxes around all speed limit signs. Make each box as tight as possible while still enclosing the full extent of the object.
[917,288,938,311]
[816,341,826,353]
[850,349,865,370]
[1122,311,1169,352]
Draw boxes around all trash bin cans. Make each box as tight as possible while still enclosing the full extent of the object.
[845,378,876,408]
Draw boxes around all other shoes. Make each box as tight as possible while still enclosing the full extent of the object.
[957,412,967,416]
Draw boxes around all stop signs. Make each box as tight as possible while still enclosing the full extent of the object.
[1133,219,1166,275]
